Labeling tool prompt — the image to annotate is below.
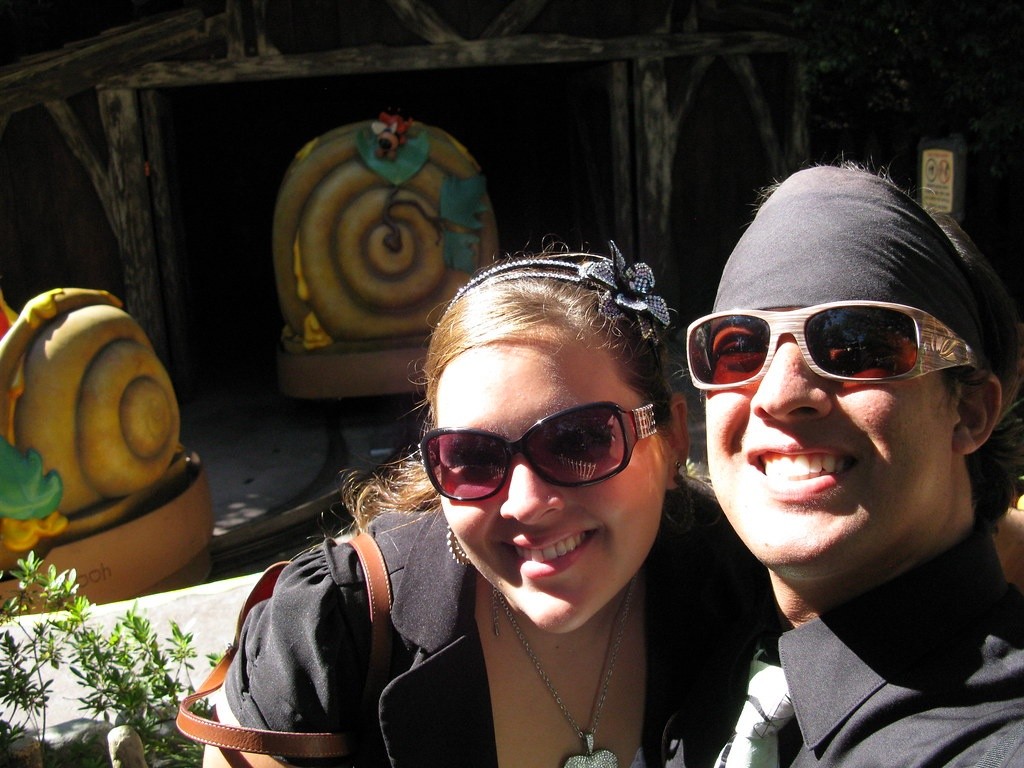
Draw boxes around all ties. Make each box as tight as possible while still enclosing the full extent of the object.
[712,647,798,768]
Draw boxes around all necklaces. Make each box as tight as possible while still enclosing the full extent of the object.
[491,574,639,767]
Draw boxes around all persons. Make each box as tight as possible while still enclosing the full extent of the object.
[202,164,1024,768]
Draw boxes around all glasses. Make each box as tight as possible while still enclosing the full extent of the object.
[414,399,659,503]
[685,299,980,391]
